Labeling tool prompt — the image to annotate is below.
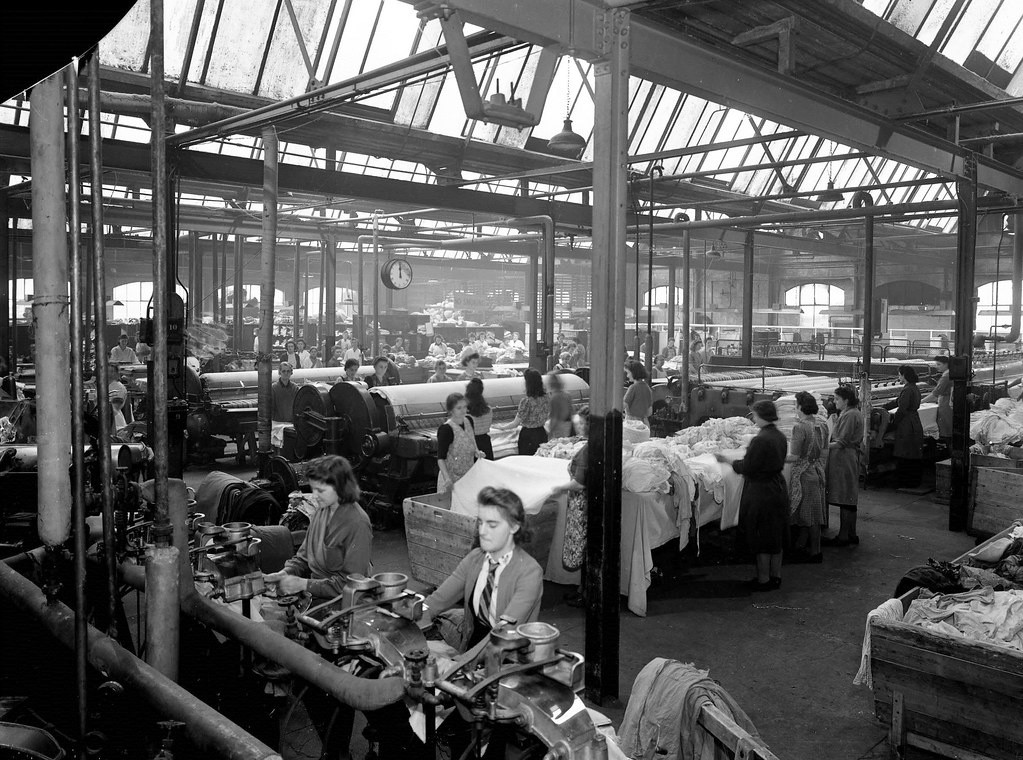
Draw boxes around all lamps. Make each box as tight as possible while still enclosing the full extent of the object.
[546,1,586,149]
[817,139,846,201]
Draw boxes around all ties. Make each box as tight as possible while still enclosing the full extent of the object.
[476,558,500,633]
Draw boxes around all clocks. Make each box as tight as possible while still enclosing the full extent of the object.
[390,260,412,287]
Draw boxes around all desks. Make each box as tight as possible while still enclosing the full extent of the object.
[550,436,830,616]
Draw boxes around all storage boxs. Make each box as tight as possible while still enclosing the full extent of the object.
[872,521,1023,760]
[966,466,1023,536]
[403,493,559,597]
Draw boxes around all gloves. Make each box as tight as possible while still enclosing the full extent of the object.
[280,574,307,595]
[264,571,288,588]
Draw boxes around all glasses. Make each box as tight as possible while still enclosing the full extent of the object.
[281,370,291,374]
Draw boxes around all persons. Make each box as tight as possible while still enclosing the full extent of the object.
[880,366,923,489]
[546,373,576,439]
[713,400,786,593]
[919,356,953,446]
[135,338,150,354]
[623,361,652,429]
[492,368,551,456]
[465,378,493,462]
[107,381,127,428]
[551,407,589,608]
[422,487,542,667]
[263,456,372,598]
[437,393,486,493]
[269,362,298,423]
[784,390,826,565]
[109,334,140,364]
[826,387,863,546]
[253,328,713,386]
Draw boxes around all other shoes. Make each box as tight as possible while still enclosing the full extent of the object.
[826,535,848,546]
[743,576,780,592]
[796,552,823,564]
[849,536,859,545]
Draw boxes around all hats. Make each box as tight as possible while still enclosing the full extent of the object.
[372,356,389,368]
